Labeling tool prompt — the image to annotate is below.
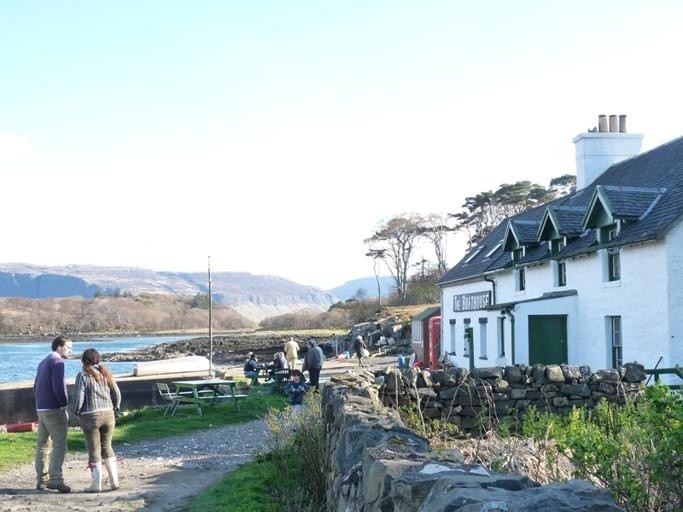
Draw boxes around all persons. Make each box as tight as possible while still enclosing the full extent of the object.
[245,336,323,391]
[32,335,71,493]
[72,349,121,491]
[286,371,309,433]
[353,335,366,368]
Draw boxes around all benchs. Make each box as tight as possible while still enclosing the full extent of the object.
[164,388,215,396]
[195,394,249,402]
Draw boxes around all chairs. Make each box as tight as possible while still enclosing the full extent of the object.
[155,382,184,418]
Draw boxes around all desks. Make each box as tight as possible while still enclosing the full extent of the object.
[171,378,241,416]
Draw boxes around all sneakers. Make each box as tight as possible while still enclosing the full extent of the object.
[47,483,71,492]
[36,481,48,489]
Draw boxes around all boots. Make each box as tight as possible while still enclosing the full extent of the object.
[83,461,101,492]
[101,456,120,490]
